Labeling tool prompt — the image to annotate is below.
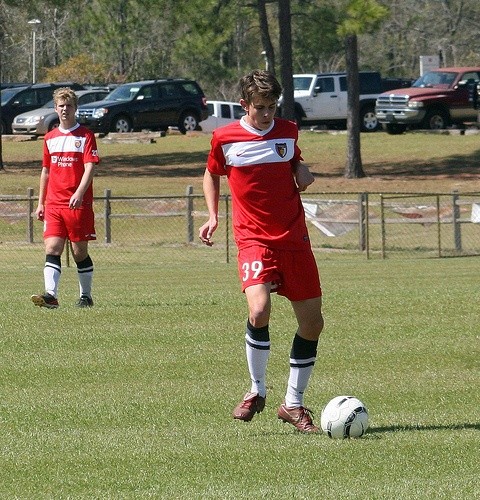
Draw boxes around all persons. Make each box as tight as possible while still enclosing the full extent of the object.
[31,87,101,308]
[199,68,324,437]
[472,72,480,130]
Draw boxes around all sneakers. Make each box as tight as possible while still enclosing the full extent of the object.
[31,293,58,309]
[77,295,93,307]
[278,400,319,433]
[233,392,266,421]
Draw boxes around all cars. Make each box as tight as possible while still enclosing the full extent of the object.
[0,82,110,135]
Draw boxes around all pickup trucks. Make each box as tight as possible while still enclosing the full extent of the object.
[199,100,246,132]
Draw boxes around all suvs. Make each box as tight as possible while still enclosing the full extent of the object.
[276,72,418,132]
[374,67,480,135]
[75,78,208,135]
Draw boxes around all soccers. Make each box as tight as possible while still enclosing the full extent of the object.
[321,395,369,440]
